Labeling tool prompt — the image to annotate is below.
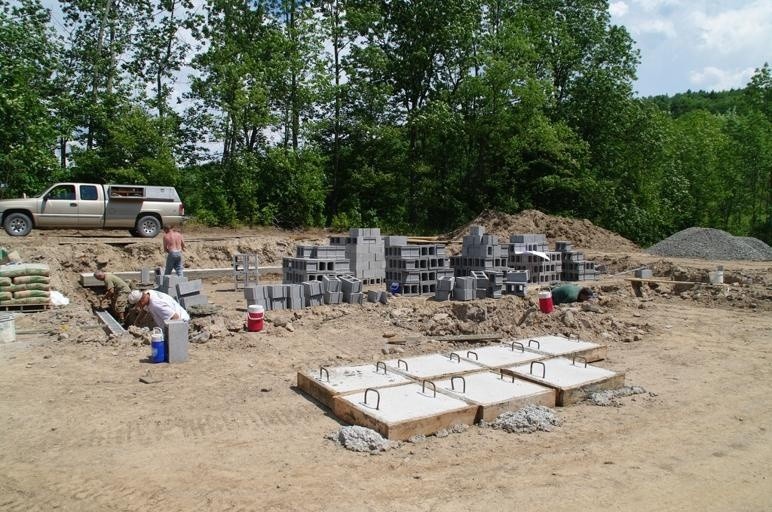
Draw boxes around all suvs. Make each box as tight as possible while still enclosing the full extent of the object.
[1,182,183,237]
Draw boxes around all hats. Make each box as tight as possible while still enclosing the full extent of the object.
[125,289,142,307]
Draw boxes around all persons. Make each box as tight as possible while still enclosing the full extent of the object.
[128,289,190,335]
[549,283,594,305]
[93,270,132,326]
[163,224,187,276]
